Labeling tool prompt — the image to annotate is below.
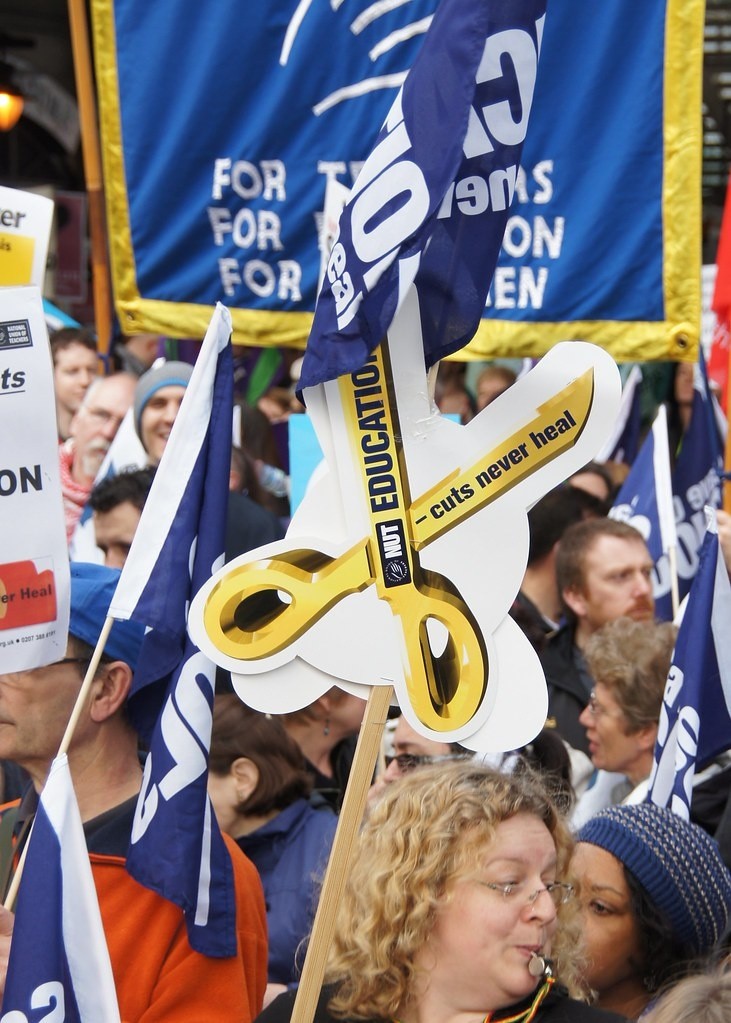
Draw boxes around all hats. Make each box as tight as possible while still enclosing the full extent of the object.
[574,803,731,955]
[133,361,194,446]
[61,561,143,668]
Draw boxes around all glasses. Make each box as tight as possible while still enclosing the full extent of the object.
[384,753,473,773]
[473,877,573,906]
[589,686,613,716]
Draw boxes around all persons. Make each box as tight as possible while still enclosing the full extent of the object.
[0,328,731,1023]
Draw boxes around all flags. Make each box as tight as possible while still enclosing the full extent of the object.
[607,405,674,622]
[672,352,726,599]
[645,506,731,821]
[296,0,547,406]
[1,751,122,1023]
[114,305,240,959]
